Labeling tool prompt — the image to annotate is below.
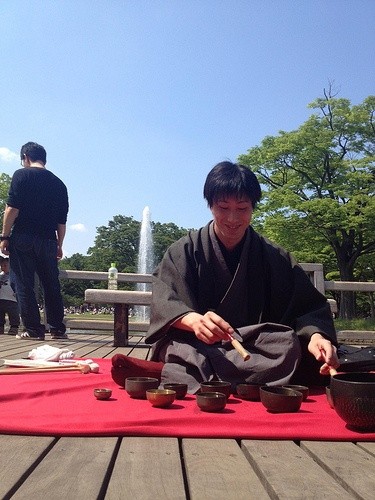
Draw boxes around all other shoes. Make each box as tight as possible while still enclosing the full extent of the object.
[0,328,4,334]
[15,331,45,341]
[7,326,19,335]
[50,331,68,338]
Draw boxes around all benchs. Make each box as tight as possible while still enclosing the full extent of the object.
[82,262,337,347]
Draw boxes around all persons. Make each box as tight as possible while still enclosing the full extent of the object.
[0,141,69,340]
[0,252,21,336]
[38,303,135,317]
[145,157,340,396]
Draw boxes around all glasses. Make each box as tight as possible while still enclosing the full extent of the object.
[21,155,25,166]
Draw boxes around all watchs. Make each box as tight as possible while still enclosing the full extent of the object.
[0,234,9,240]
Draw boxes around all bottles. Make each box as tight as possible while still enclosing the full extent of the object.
[108,263,118,290]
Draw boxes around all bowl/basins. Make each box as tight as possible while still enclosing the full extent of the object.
[145,389,176,408]
[237,382,265,401]
[283,384,309,402]
[163,383,188,400]
[330,373,375,432]
[258,386,303,413]
[125,377,159,399]
[196,392,226,412]
[326,385,334,409]
[94,388,112,400]
[199,381,233,401]
